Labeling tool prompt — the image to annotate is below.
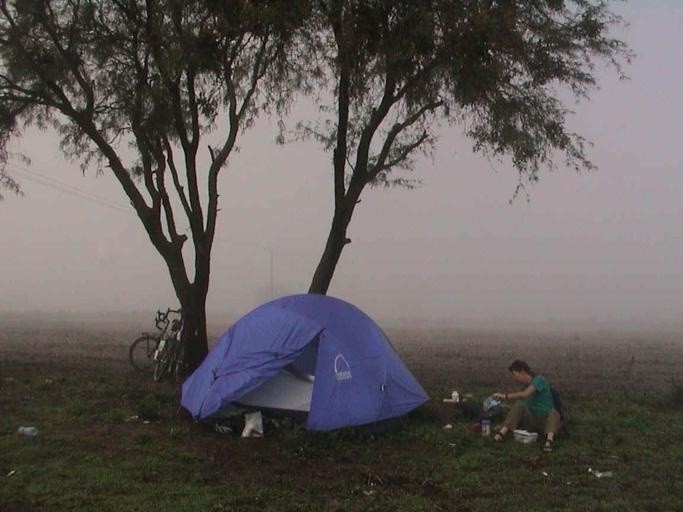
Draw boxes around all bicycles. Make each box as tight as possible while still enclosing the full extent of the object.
[128,307,199,385]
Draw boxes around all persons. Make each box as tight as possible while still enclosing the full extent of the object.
[491,359,566,454]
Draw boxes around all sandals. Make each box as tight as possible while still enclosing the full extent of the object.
[494,432,505,442]
[543,437,555,453]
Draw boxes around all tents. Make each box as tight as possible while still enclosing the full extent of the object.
[176,292,430,432]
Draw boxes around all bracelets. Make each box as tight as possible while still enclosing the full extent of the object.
[504,392,509,399]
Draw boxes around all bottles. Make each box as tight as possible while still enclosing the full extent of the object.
[481,419,491,437]
[451,390,459,403]
[17,426,38,438]
